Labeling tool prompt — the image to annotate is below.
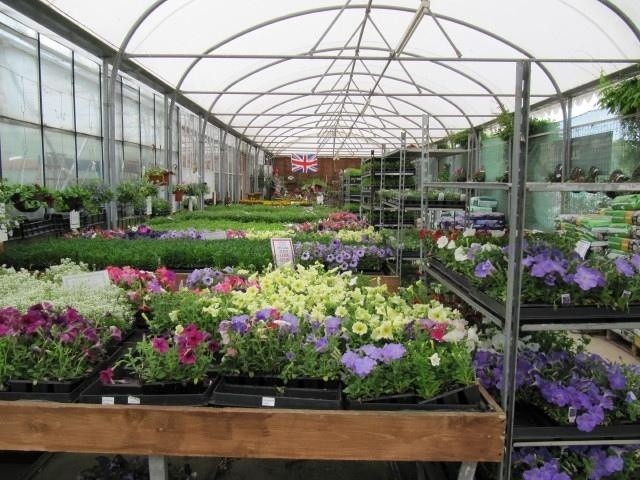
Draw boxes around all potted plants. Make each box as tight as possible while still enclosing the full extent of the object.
[336,148,465,261]
[0,166,209,217]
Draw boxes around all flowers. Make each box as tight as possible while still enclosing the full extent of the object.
[0,210,640,480]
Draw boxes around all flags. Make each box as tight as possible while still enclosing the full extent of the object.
[291,153,319,174]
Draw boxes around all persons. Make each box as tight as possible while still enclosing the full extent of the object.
[312,185,324,205]
